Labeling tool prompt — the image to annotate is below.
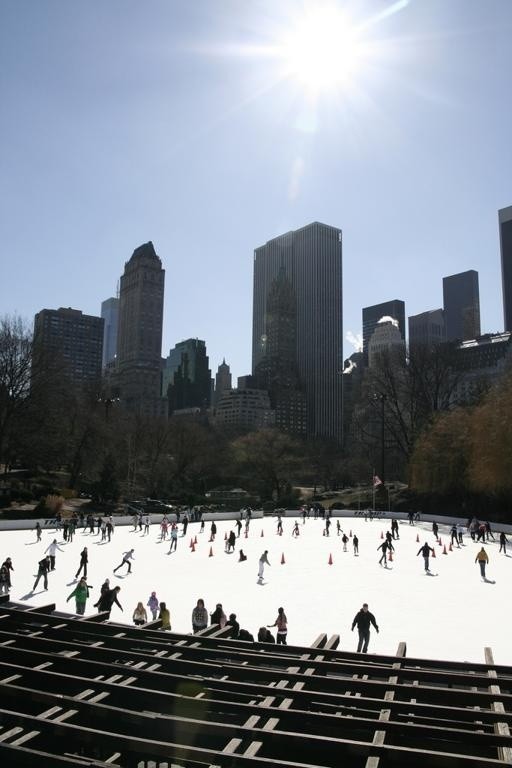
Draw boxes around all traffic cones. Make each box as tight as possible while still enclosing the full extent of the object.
[449,542,453,551]
[431,546,437,557]
[442,544,447,554]
[349,529,352,536]
[389,550,394,561]
[381,531,384,537]
[188,527,333,565]
[416,534,420,541]
[439,537,443,545]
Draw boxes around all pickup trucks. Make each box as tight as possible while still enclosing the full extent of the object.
[129,498,173,515]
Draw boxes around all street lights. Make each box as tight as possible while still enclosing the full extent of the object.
[94,391,121,416]
[373,391,388,491]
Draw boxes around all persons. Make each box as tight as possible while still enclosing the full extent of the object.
[301,503,332,521]
[258,550,270,577]
[378,520,399,564]
[235,504,252,537]
[133,592,288,645]
[226,530,236,551]
[161,506,218,551]
[408,508,420,524]
[433,516,509,577]
[351,603,379,653]
[417,542,433,571]
[365,511,380,521]
[0,509,151,615]
[325,517,358,555]
[273,504,299,536]
